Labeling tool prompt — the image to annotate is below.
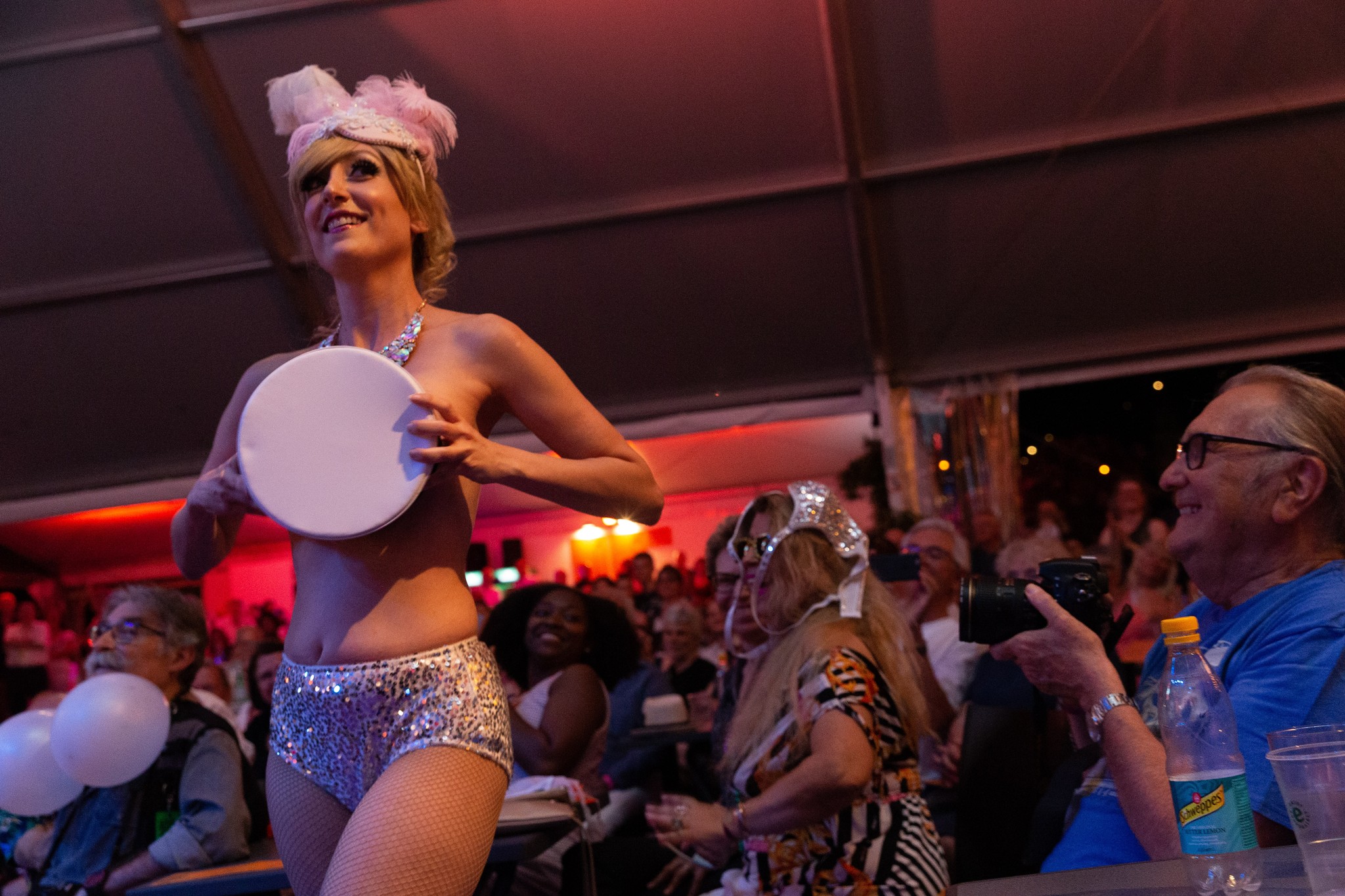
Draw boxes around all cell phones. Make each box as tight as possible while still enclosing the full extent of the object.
[867,552,920,581]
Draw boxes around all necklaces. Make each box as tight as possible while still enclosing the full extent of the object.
[318,296,430,367]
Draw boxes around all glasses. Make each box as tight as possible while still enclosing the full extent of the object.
[1176,432,1305,470]
[736,533,769,559]
[89,620,165,644]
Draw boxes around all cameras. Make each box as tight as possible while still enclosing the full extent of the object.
[959,554,1111,648]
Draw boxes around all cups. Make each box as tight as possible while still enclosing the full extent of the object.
[1262,744,1345,895]
[1267,724,1345,776]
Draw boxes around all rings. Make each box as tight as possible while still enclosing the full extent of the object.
[673,814,685,830]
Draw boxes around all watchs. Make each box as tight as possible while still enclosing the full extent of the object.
[1083,693,1132,744]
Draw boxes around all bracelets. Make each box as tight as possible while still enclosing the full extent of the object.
[733,801,755,837]
[721,809,738,841]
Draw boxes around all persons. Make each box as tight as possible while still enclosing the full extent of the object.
[170,60,663,896]
[1,362,1344,896]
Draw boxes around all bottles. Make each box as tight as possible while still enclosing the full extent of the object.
[1159,614,1269,894]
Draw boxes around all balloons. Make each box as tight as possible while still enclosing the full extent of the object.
[0,710,83,813]
[49,672,172,789]
